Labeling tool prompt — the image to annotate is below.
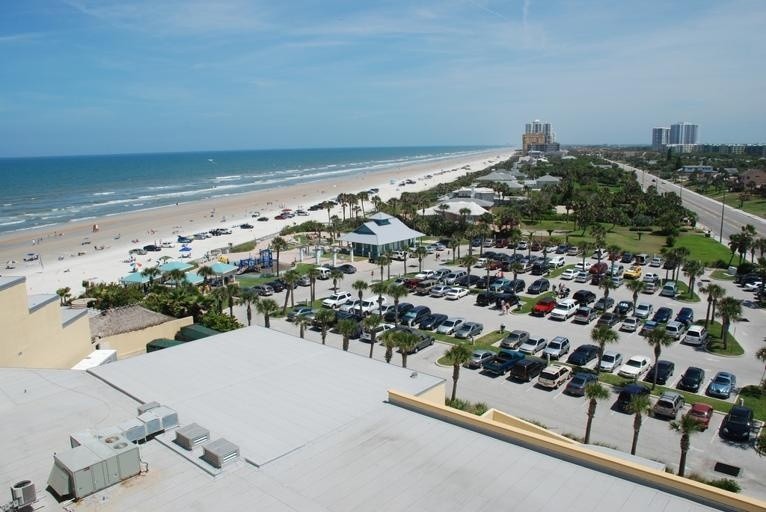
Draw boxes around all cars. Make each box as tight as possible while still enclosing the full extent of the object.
[686,402,713,431]
[274,162,471,220]
[24,251,38,262]
[127,216,269,255]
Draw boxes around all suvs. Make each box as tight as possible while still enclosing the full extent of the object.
[616,381,651,414]
[721,405,754,441]
[653,389,685,419]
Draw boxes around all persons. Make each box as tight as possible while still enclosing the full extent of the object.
[500,300,510,315]
[495,271,504,277]
[552,283,566,298]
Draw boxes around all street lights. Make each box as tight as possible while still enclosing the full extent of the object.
[620,158,733,245]
